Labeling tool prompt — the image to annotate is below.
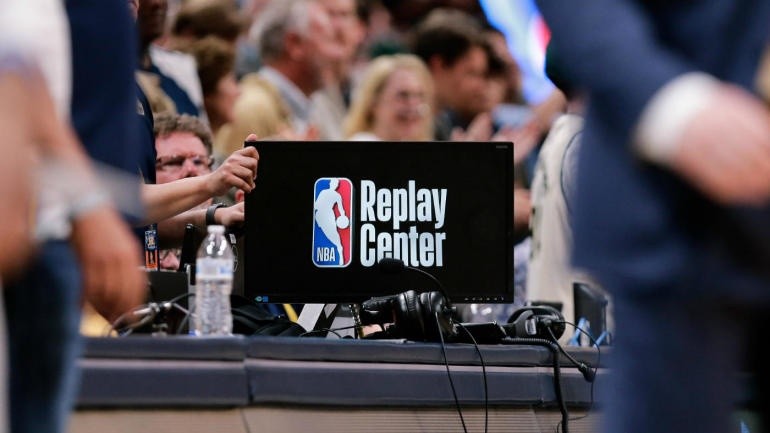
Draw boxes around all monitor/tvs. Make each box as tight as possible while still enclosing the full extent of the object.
[243,141,514,304]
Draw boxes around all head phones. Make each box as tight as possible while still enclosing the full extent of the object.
[452,305,566,344]
[363,289,457,343]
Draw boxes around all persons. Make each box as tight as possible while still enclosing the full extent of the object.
[136,0,565,224]
[531,0,770,433]
[0,0,149,433]
[153,112,244,245]
[343,53,436,142]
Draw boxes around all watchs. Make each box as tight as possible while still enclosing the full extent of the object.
[206,202,227,225]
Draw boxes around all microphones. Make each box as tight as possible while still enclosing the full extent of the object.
[547,327,596,383]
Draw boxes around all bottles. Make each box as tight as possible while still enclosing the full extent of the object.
[194,225,235,337]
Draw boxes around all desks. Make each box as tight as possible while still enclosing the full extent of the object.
[71,334,608,433]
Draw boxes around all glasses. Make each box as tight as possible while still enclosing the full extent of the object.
[156,155,215,170]
